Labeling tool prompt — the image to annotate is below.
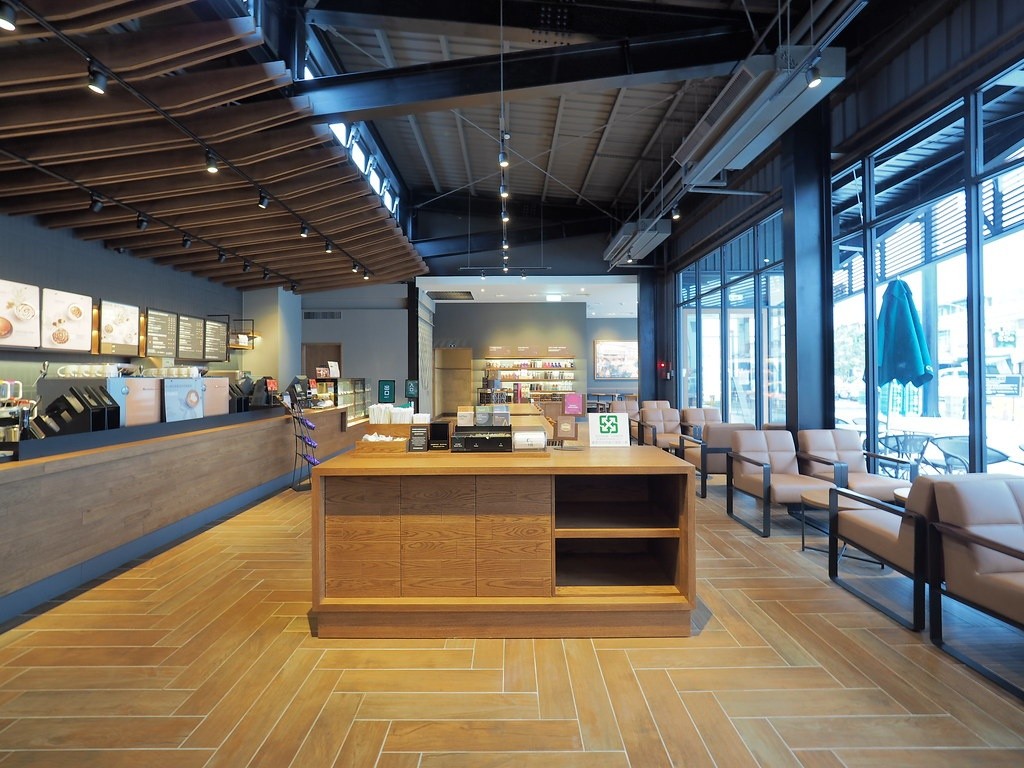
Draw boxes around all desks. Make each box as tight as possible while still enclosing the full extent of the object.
[606,393,621,401]
[669,441,701,457]
[893,487,911,508]
[799,489,884,570]
[592,393,606,407]
[625,395,637,401]
[509,415,561,446]
[481,404,541,416]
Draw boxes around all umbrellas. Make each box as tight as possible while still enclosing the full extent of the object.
[863,279,934,455]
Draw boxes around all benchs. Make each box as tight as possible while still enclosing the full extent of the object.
[587,388,637,413]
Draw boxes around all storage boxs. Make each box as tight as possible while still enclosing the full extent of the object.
[451,432,512,453]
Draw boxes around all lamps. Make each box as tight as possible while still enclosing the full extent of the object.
[500,185,508,198]
[501,211,509,222]
[182,239,192,249]
[502,251,508,260]
[363,272,370,280]
[503,263,508,272]
[301,226,309,238]
[325,243,332,253]
[672,209,680,219]
[499,152,509,167]
[805,67,822,88]
[205,157,218,173]
[502,240,509,249]
[258,195,269,210]
[89,199,104,214]
[136,219,148,231]
[626,255,632,263]
[217,254,297,291]
[87,73,107,95]
[352,264,359,273]
[0,2,17,31]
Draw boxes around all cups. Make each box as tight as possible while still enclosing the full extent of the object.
[185,389,200,408]
[57,365,117,377]
[142,369,156,377]
[169,368,198,378]
[152,369,168,377]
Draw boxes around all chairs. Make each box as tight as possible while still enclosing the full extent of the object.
[862,434,942,478]
[928,478,1024,700]
[929,435,1024,475]
[638,408,698,453]
[679,423,756,499]
[629,408,653,446]
[682,407,722,440]
[795,429,918,529]
[828,474,1020,633]
[726,430,842,538]
[611,400,640,439]
[642,400,670,408]
[761,423,786,430]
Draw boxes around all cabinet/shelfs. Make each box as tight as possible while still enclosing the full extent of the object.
[316,377,367,423]
[312,445,697,638]
[485,356,577,402]
